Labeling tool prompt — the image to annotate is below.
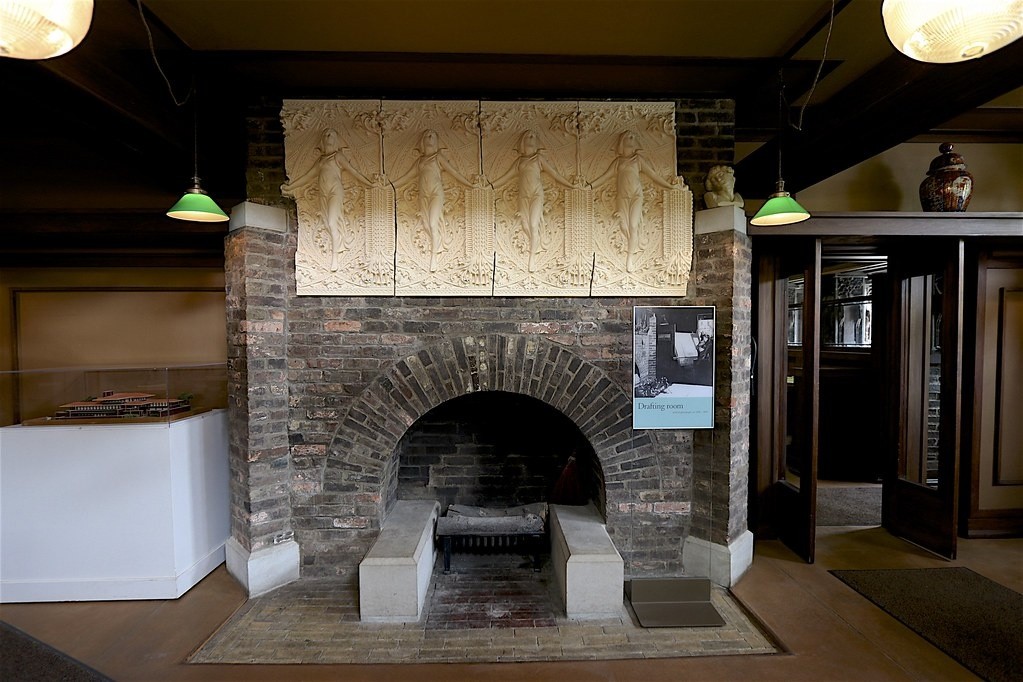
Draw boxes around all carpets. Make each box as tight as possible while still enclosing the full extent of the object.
[0,621,116,682]
[826,567,1023,682]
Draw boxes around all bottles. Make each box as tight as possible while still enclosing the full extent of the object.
[919,143,975,212]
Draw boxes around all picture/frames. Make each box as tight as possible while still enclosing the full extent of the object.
[632,304,715,429]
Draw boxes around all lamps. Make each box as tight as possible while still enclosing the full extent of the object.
[167,106,231,224]
[0,0,98,59]
[884,0,1023,63]
[753,67,810,226]
